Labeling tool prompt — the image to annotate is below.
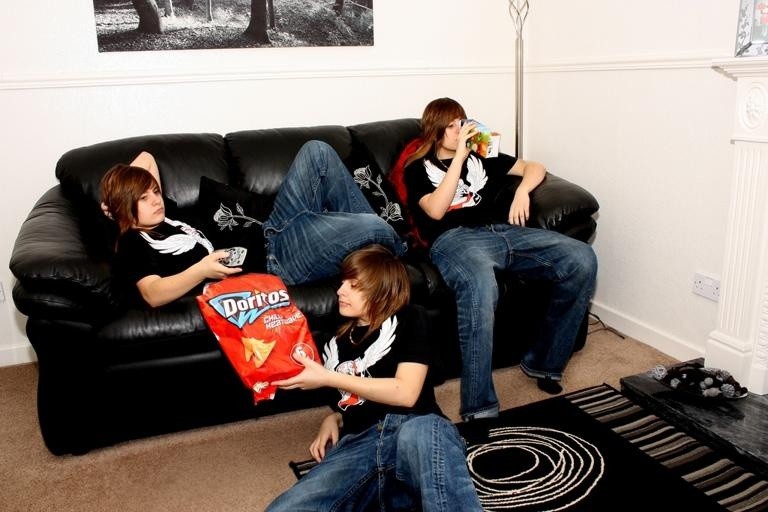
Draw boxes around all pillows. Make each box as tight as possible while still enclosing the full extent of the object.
[344,142,409,236]
[194,175,276,232]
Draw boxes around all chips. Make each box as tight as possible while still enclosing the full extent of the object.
[239,336,276,368]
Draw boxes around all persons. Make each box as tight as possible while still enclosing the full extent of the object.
[403,97,597,443]
[98,140,408,310]
[261,242,482,512]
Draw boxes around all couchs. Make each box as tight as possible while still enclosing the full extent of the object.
[9,119,600,457]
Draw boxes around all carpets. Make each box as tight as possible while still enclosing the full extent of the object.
[288,381,768,511]
